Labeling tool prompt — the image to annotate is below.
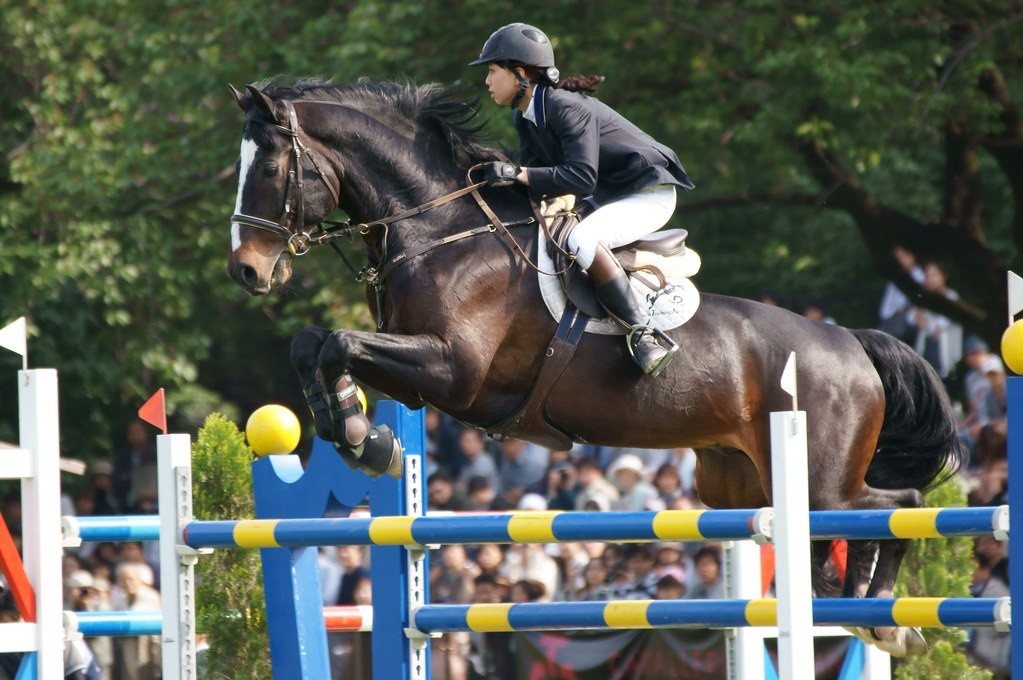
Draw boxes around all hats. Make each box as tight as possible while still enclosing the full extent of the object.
[983,356,1003,374]
[659,540,685,551]
[607,454,647,479]
[654,565,685,586]
[965,335,988,355]
[516,492,546,509]
[65,569,94,587]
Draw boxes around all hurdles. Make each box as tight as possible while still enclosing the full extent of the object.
[0,373,1023,680]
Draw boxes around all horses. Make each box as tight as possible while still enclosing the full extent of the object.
[964,415,1009,598]
[227,72,967,659]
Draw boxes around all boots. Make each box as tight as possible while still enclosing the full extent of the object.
[600,273,671,373]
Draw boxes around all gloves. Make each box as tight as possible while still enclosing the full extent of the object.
[482,161,522,186]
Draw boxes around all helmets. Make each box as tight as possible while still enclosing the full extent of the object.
[467,22,554,68]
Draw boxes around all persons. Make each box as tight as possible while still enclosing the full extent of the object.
[799,238,1010,599]
[473,22,677,373]
[319,409,728,680]
[0,417,163,680]
